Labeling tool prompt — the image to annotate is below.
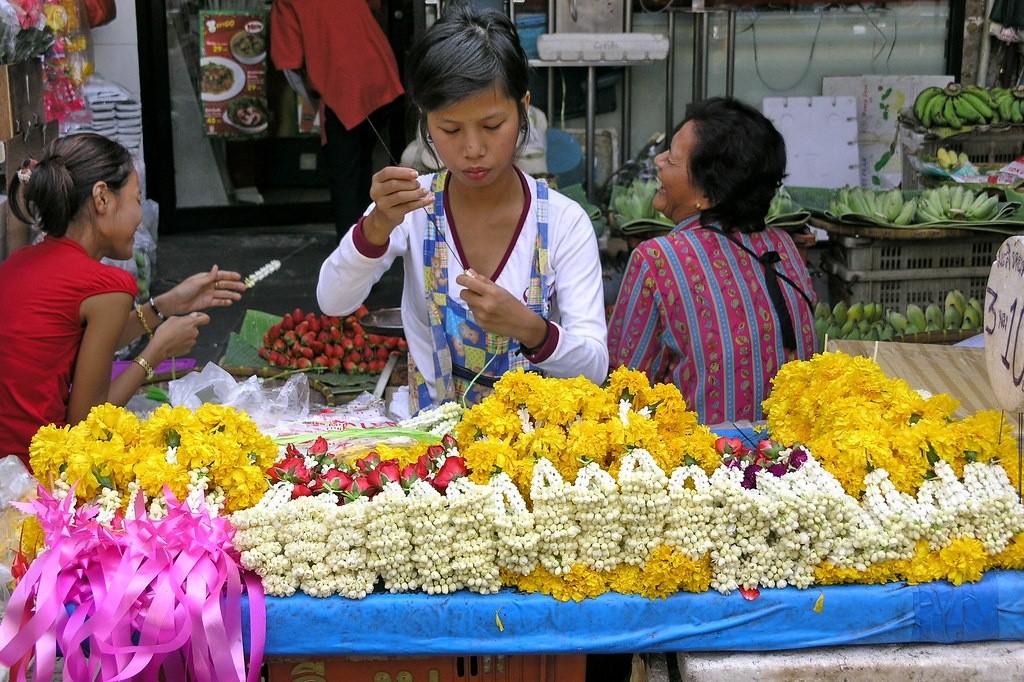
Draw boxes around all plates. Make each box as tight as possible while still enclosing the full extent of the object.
[199,56,247,102]
[232,52,267,65]
[359,307,404,337]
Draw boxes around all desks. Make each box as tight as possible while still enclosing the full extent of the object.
[239,570,1024,653]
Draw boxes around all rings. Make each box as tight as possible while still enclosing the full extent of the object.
[215,282,218,288]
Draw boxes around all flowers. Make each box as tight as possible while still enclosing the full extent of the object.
[0,0,96,80]
[1,363,1024,604]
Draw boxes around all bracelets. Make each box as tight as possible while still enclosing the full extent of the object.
[150,297,166,321]
[136,304,153,339]
[521,317,548,351]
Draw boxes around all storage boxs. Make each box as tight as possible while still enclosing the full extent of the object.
[644,638,1024,682]
[266,652,586,682]
[536,31,670,60]
[827,338,1024,447]
[560,112,1024,344]
[516,11,546,60]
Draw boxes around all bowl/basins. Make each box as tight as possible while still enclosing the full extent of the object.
[221,108,269,135]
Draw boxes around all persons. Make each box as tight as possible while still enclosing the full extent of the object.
[604,96,818,426]
[271,0,404,304]
[0,133,247,476]
[315,7,609,418]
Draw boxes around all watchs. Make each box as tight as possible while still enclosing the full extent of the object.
[134,356,154,380]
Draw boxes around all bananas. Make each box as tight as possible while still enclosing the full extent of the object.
[611,173,791,229]
[827,183,1002,224]
[913,80,1024,174]
[814,287,984,344]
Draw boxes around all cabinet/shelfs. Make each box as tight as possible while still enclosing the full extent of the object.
[0,58,43,141]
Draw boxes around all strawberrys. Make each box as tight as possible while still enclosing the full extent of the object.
[258,301,408,376]
